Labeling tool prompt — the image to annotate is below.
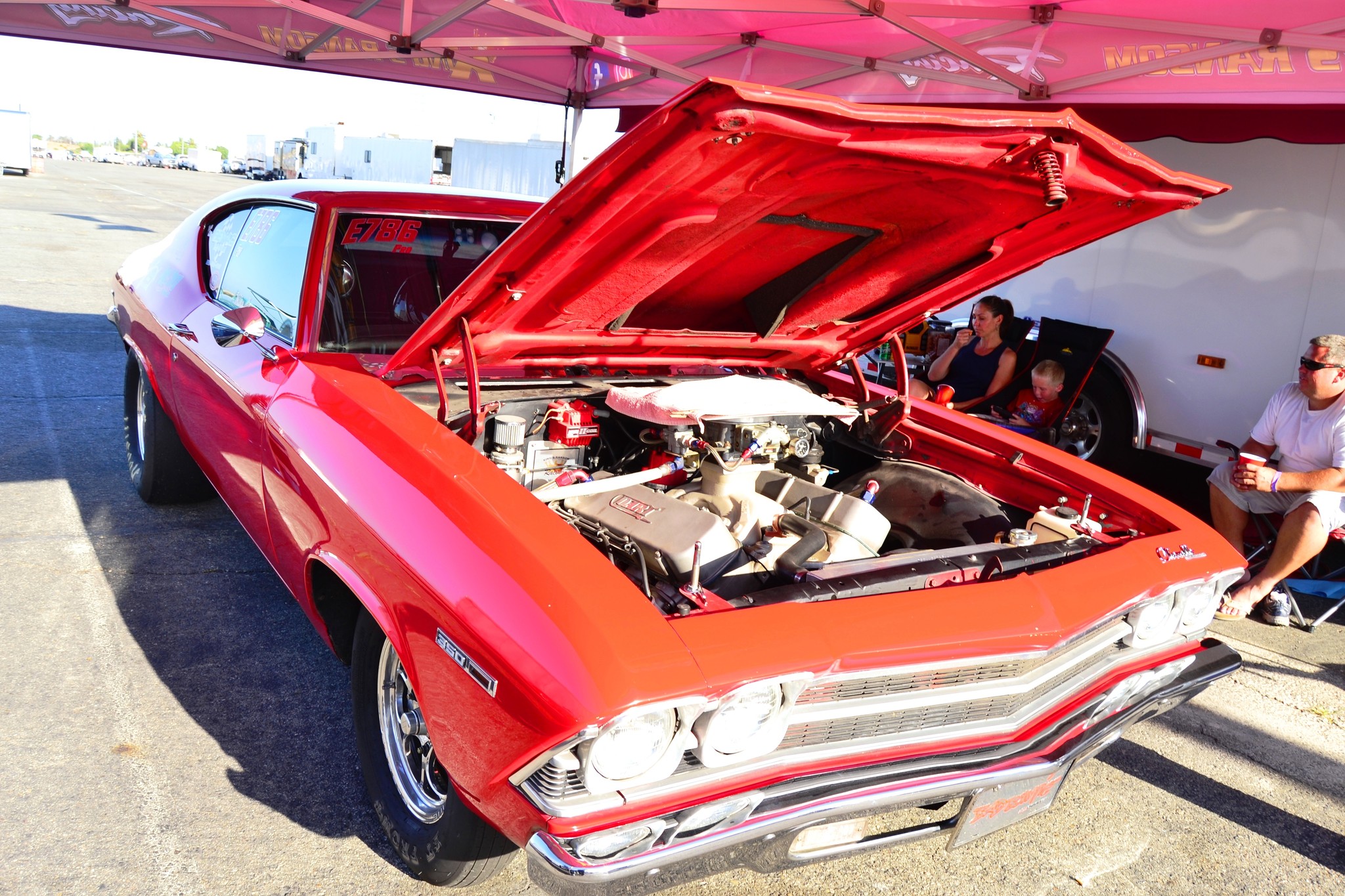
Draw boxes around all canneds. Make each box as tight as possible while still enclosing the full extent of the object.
[879,342,891,361]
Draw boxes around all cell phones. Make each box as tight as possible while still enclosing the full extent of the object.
[993,405,1017,421]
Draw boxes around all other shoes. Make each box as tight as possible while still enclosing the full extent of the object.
[1262,586,1292,626]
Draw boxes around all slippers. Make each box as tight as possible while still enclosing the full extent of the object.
[1213,590,1254,621]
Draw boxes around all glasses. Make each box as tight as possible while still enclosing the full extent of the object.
[1300,356,1344,371]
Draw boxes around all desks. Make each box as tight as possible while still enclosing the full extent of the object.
[864,350,930,384]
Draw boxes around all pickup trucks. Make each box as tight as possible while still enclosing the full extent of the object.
[147,152,176,169]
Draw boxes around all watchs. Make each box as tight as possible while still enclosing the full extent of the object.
[946,401,954,410]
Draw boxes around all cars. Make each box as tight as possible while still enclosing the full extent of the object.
[95,152,147,166]
[176,154,189,170]
[103,79,1248,896]
[32,144,94,162]
[245,167,265,180]
[265,169,286,181]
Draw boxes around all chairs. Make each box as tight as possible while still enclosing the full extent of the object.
[1216,440,1345,633]
[893,304,1035,389]
[962,316,1114,446]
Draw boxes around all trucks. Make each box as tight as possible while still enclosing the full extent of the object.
[846,138,1345,541]
[0,109,31,176]
[188,149,223,172]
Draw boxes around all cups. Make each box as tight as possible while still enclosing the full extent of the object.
[936,384,955,408]
[1240,452,1267,472]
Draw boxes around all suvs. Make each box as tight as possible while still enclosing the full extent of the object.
[222,158,246,175]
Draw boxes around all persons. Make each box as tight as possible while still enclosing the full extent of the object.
[966,358,1068,441]
[906,294,1017,412]
[1208,335,1345,622]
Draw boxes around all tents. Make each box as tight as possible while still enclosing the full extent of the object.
[0,0,1344,197]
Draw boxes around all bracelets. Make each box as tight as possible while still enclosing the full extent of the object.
[1270,471,1282,493]
